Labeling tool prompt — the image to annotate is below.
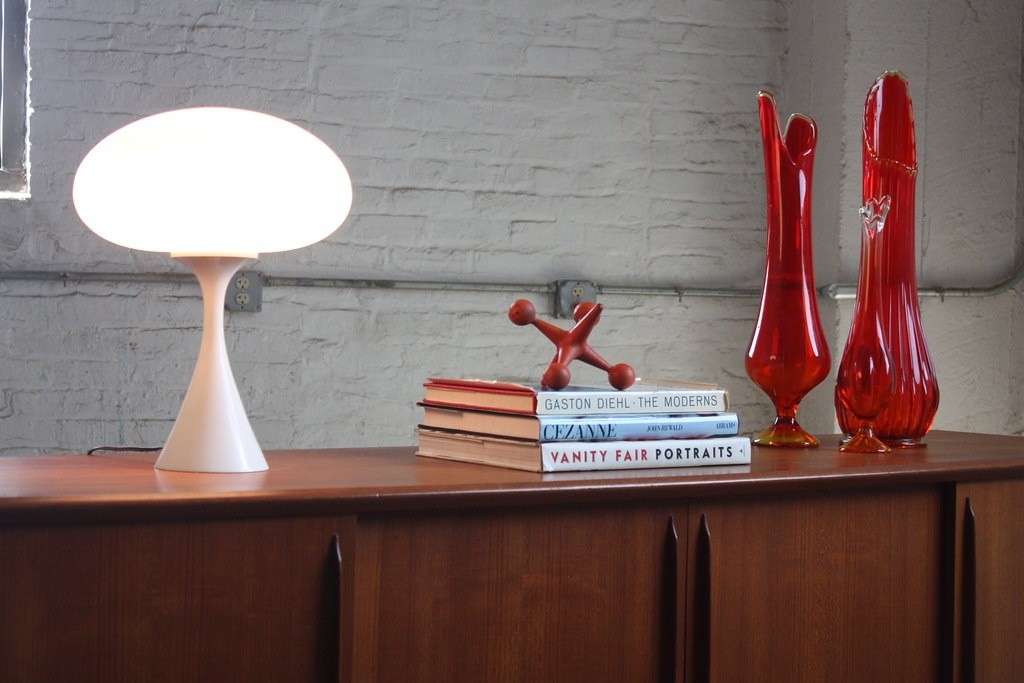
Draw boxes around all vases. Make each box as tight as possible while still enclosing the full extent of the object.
[742,89,831,450]
[834,69,940,452]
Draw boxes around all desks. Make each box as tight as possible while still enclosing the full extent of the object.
[0,431,1024,683]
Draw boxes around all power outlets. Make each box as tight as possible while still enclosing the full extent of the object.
[553,280,596,320]
[225,271,263,312]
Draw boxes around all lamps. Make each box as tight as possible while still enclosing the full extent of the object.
[71,107,354,473]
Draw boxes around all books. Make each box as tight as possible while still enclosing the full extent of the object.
[413,402,753,473]
[423,376,730,415]
